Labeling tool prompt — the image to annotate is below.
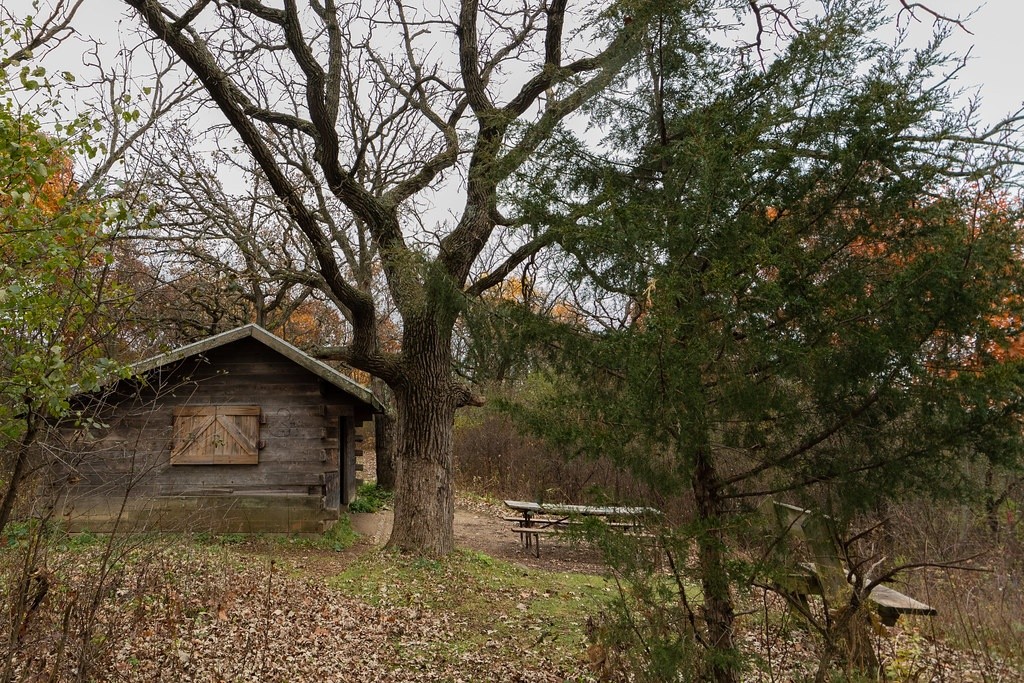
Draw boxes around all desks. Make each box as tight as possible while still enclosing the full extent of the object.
[503,499,666,555]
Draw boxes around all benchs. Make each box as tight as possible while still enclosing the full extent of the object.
[511,527,670,559]
[771,499,938,629]
[500,516,645,547]
